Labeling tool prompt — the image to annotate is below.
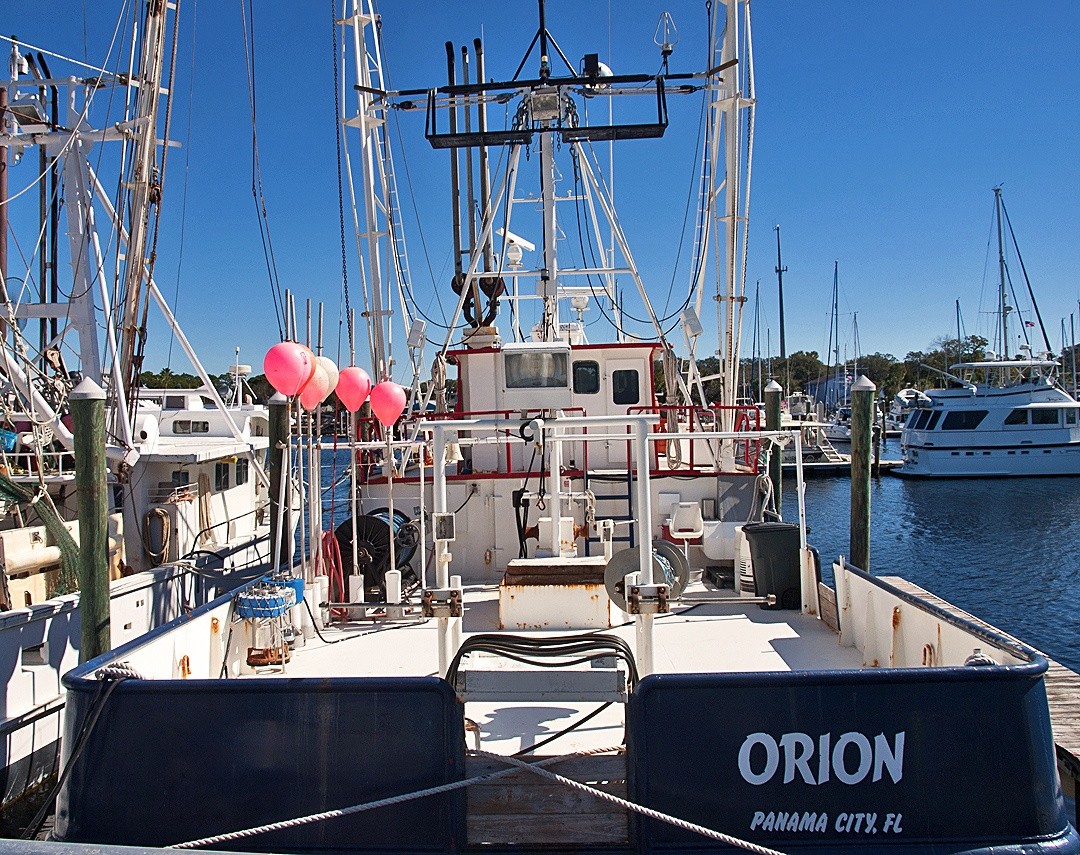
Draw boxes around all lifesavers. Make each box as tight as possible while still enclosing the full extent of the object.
[805,455,815,463]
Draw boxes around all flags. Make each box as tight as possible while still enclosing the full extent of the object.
[1025,320,1035,327]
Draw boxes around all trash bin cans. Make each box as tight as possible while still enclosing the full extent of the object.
[741,522,811,610]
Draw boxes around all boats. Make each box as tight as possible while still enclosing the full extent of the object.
[1,0,1080,855]
[888,182,1080,480]
